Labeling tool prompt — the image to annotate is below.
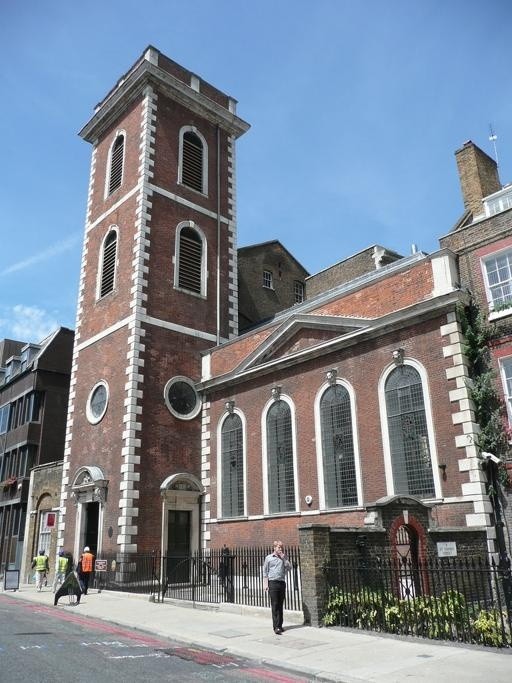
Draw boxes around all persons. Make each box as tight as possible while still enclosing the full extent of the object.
[262,540,293,634]
[31,545,95,595]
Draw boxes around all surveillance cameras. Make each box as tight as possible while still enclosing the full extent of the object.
[482,452,491,459]
[488,453,502,466]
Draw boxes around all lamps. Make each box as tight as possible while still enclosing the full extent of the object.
[325,370,337,383]
[271,385,281,400]
[390,348,404,366]
[225,400,233,412]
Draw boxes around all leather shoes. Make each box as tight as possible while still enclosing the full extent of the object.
[274,626,283,634]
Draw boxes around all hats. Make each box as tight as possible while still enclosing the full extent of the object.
[59,551,64,556]
[39,550,45,555]
[84,546,90,552]
